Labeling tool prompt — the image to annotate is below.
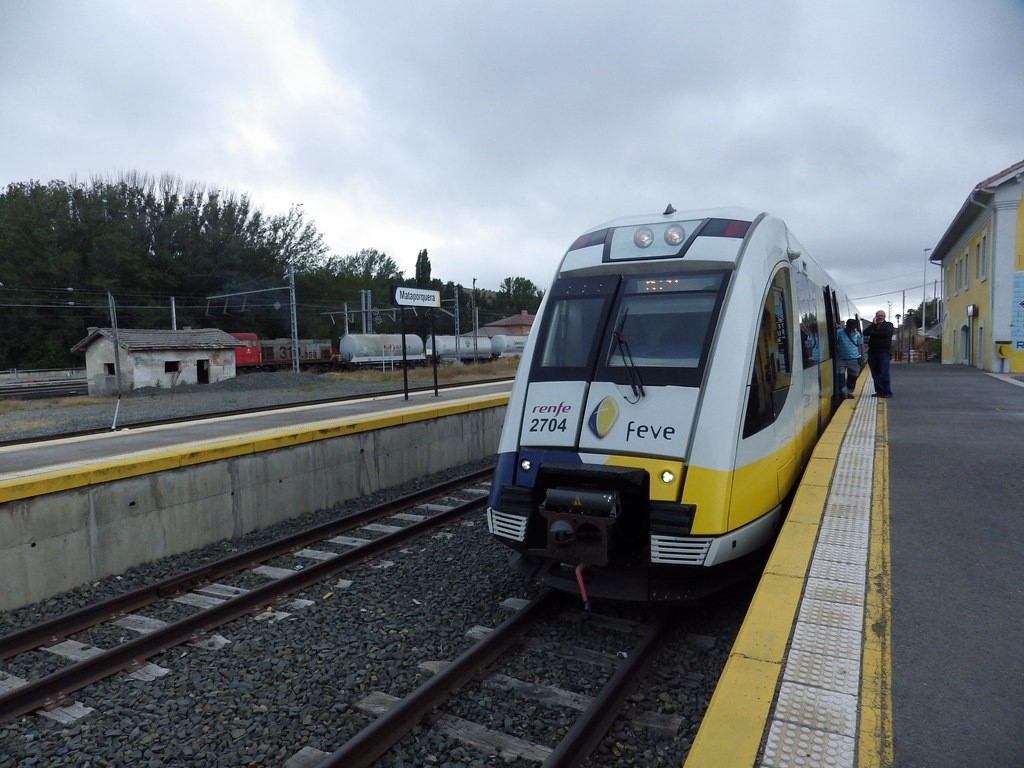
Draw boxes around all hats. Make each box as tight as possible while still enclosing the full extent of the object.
[845,319,856,328]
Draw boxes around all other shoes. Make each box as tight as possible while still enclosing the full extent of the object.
[880,393,891,398]
[871,392,881,397]
[848,393,855,399]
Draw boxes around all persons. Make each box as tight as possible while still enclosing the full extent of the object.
[863,310,895,398]
[835,319,862,399]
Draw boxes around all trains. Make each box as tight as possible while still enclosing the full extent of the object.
[486,203,864,615]
[228,330,532,372]
